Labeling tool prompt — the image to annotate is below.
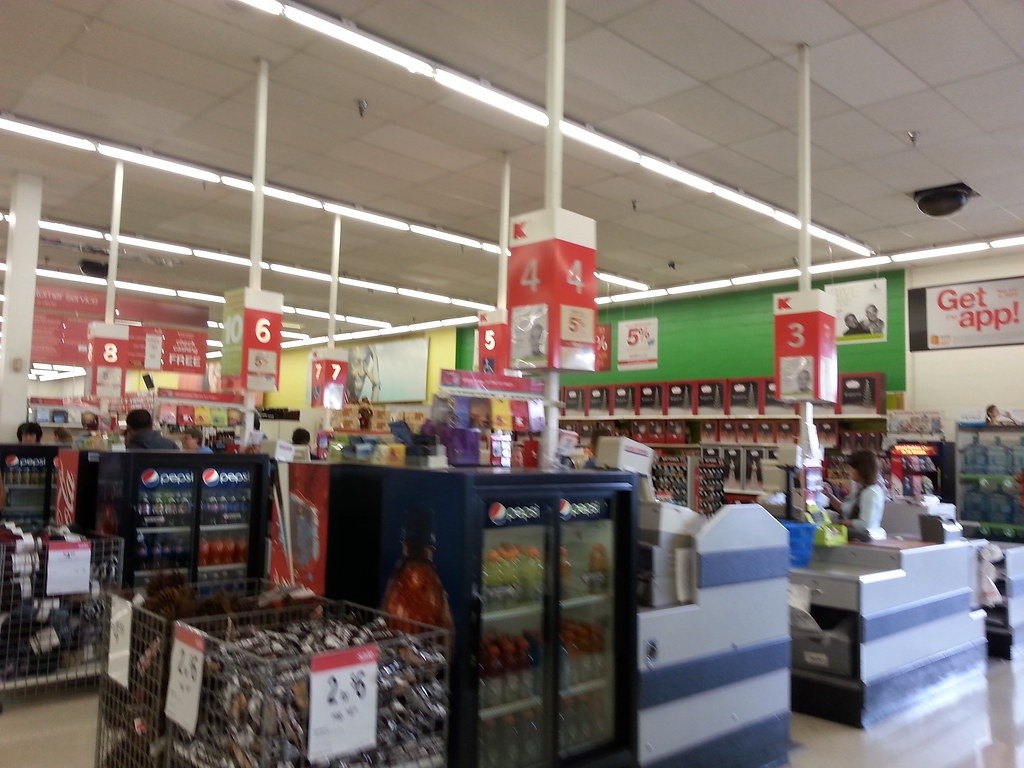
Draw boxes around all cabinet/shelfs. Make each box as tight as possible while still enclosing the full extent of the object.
[956,419,1024,543]
[560,414,889,517]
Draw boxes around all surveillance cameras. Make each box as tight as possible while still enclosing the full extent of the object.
[78,260,109,277]
[914,183,973,217]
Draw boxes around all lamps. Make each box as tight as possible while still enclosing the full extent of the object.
[0,0,1024,382]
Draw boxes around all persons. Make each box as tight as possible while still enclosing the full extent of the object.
[238,410,268,446]
[482,358,494,373]
[17,421,43,445]
[124,408,180,451]
[530,325,543,356]
[181,427,214,453]
[54,427,73,444]
[344,343,381,404]
[843,304,884,335]
[74,412,112,444]
[292,427,320,460]
[584,427,611,470]
[984,405,1019,426]
[797,370,810,391]
[823,448,885,541]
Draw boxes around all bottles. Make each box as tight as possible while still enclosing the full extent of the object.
[133,489,253,599]
[4,466,58,488]
[962,432,1023,527]
[480,540,616,768]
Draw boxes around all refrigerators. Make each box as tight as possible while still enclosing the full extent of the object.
[267,458,641,768]
[53,448,271,604]
[2,441,60,533]
